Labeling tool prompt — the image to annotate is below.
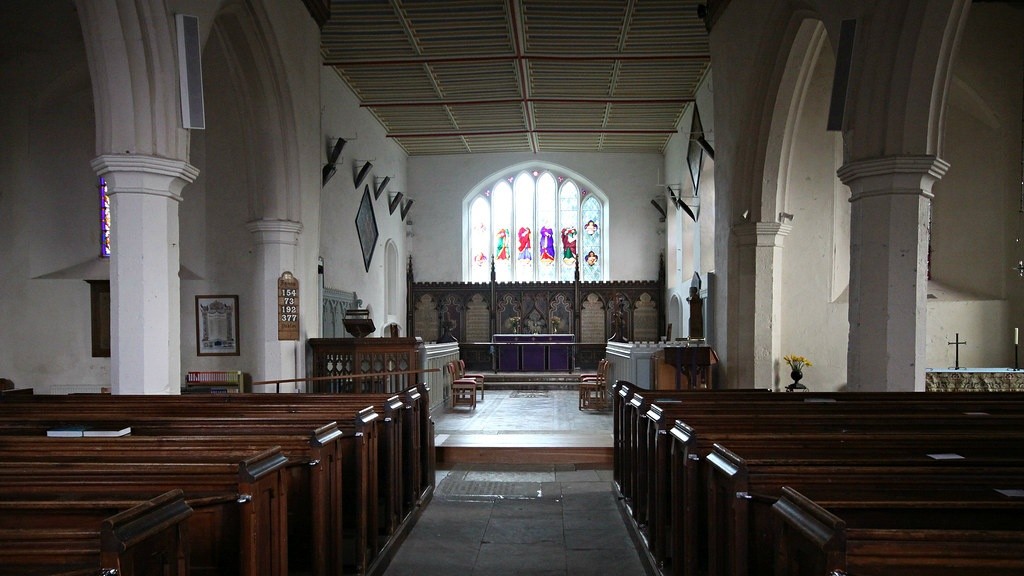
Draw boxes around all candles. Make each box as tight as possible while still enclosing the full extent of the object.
[1015,328,1018,344]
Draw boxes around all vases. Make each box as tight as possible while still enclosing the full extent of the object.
[513,326,517,333]
[553,324,557,332]
[789,369,804,386]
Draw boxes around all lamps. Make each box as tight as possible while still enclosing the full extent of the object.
[780,212,794,223]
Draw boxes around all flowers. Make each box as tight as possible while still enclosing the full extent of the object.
[509,317,521,325]
[783,354,813,369]
[551,316,560,324]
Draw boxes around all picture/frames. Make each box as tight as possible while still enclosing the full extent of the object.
[195,295,240,356]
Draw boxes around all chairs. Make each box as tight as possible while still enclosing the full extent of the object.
[459,359,485,400]
[581,361,609,408]
[448,363,476,411]
[579,359,605,410]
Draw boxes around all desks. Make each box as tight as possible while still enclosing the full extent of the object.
[0,382,436,576]
[490,334,576,374]
[927,367,1024,391]
[612,379,1024,576]
